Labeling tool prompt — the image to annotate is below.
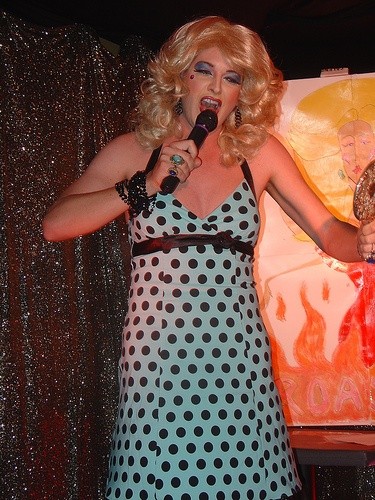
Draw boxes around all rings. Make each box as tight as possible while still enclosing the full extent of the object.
[366,253,375,264]
[169,167,179,175]
[371,244,374,254]
[169,154,184,166]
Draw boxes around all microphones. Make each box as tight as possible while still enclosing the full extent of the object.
[161,109,217,194]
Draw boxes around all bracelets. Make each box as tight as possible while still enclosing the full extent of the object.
[115,171,155,216]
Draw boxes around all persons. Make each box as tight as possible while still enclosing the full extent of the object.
[41,17,375,500]
